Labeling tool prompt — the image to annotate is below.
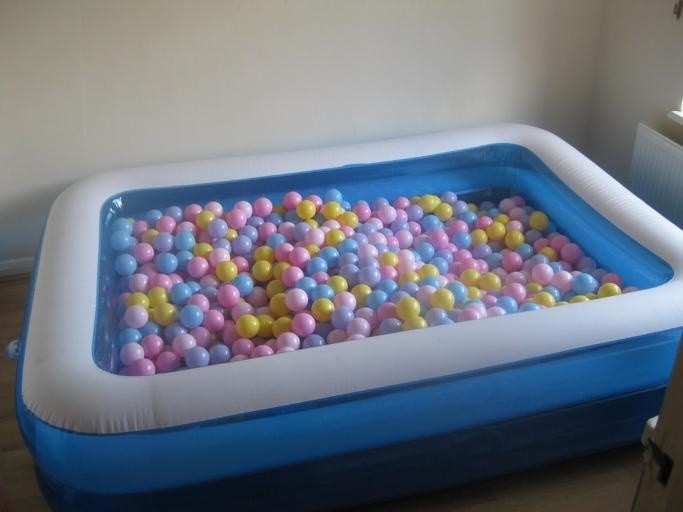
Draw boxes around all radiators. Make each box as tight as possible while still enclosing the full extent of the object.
[626,121,682,230]
